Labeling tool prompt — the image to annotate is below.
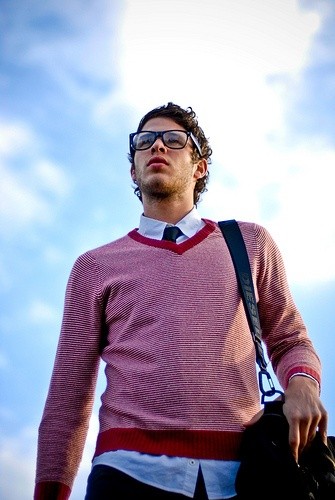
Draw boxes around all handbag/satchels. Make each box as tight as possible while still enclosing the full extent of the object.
[235,402,335,500]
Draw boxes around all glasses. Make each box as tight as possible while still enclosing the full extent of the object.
[129,130,204,159]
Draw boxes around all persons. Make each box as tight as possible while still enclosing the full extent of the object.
[35,100,330,499]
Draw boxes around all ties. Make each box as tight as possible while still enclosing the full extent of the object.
[162,226,183,244]
